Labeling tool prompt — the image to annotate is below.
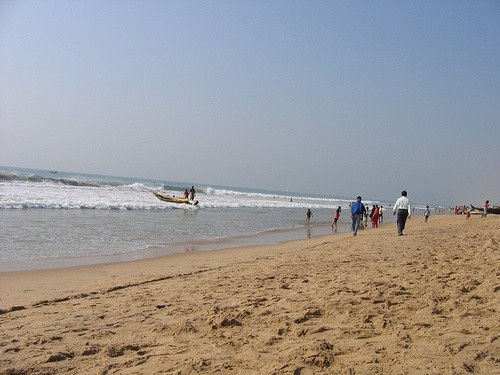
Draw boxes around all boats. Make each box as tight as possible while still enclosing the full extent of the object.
[473,206,500,214]
[152,191,199,205]
[470,210,483,215]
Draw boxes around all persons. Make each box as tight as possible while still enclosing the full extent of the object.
[424,206,430,223]
[331,206,341,227]
[349,196,384,236]
[393,191,411,236]
[184,186,196,200]
[305,209,313,221]
[449,199,489,218]
